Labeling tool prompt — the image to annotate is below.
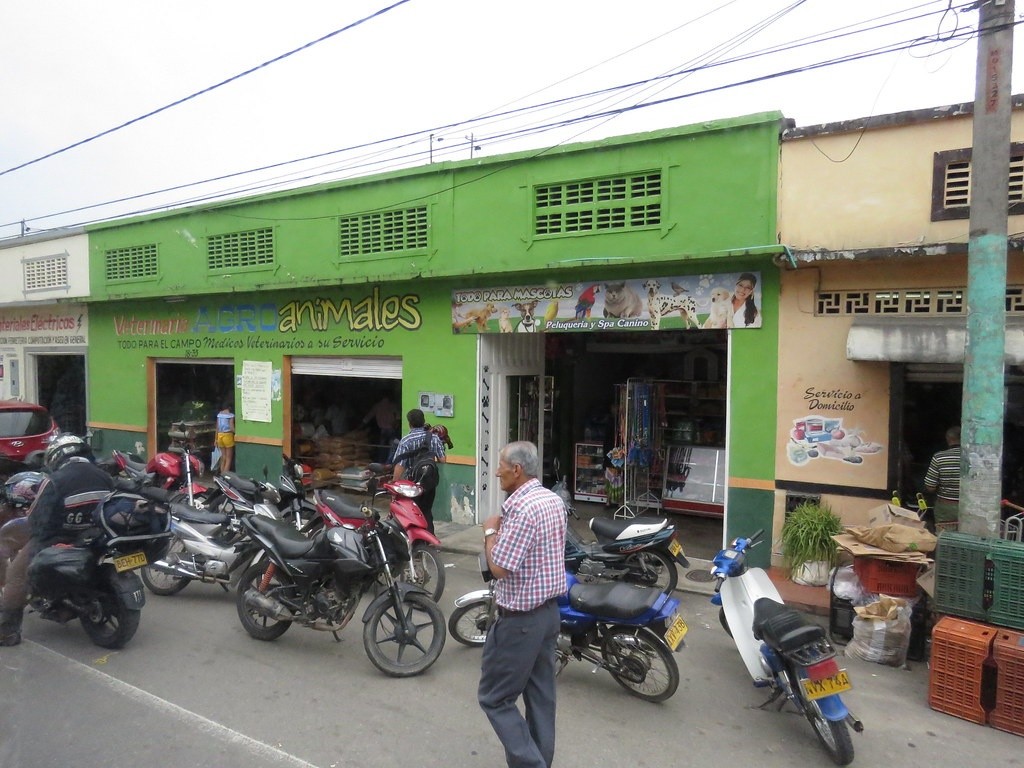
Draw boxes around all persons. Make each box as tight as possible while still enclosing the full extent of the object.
[298,392,355,435]
[1003,423,1024,497]
[452,296,478,333]
[477,441,567,768]
[214,403,236,472]
[702,273,761,327]
[391,409,446,562]
[0,433,114,647]
[924,426,960,523]
[360,392,400,446]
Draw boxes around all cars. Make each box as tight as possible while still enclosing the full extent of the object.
[0,400,61,461]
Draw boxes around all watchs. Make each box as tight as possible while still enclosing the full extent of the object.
[485,528,498,536]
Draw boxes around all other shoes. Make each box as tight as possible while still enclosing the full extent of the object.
[0,630,21,646]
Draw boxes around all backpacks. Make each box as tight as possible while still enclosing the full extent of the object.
[393,431,439,495]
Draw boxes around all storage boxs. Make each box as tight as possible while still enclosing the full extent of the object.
[928,528,1024,740]
[853,556,922,597]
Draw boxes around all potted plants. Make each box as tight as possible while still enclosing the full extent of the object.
[776,496,843,587]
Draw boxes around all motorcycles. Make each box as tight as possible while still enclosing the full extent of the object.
[448,539,688,702]
[0,430,446,677]
[710,528,864,765]
[549,455,691,595]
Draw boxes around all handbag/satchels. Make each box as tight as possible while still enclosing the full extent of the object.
[91,489,173,541]
[210,446,223,471]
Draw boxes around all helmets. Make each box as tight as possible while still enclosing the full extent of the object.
[43,435,93,473]
[7,471,47,506]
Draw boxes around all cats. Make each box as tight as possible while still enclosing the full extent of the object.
[603,282,643,319]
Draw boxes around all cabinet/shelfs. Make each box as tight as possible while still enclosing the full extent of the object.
[170,419,217,455]
[575,442,725,519]
[616,381,726,447]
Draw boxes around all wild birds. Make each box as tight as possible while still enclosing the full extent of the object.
[543,298,559,330]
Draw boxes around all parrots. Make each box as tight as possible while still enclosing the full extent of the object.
[575,282,601,323]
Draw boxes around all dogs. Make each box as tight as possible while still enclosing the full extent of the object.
[642,279,703,329]
[703,286,734,329]
[498,309,512,332]
[465,303,497,334]
[512,300,539,332]
